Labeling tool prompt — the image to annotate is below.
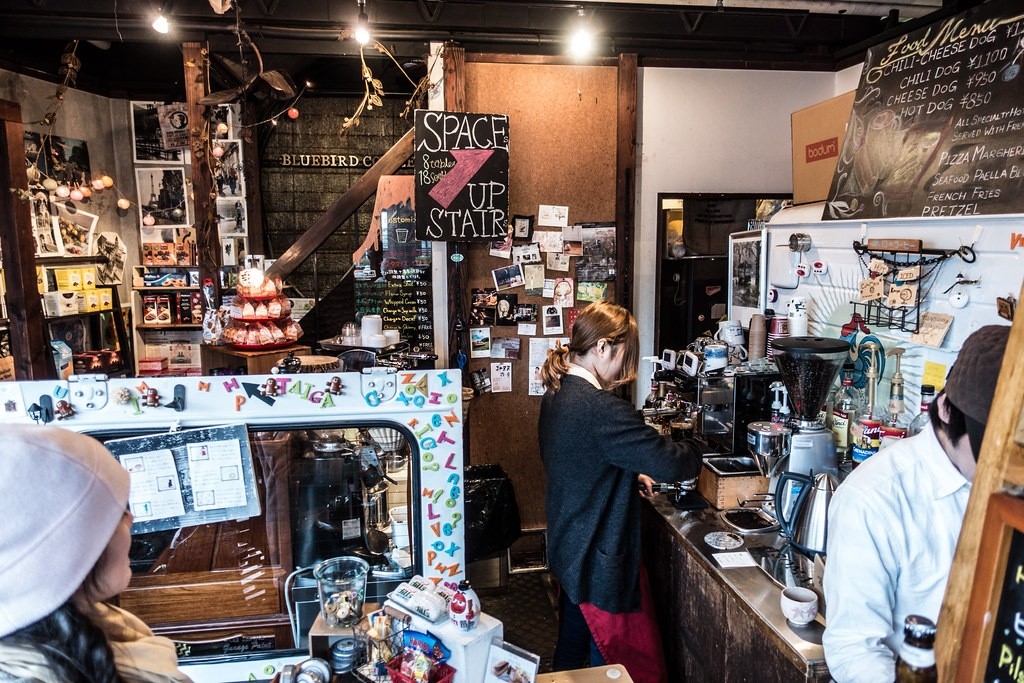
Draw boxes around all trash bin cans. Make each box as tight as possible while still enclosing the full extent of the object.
[464,462,508,596]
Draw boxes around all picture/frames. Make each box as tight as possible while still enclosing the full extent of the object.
[129,100,185,165]
[134,167,191,229]
[213,140,246,198]
[216,197,249,237]
[209,103,233,140]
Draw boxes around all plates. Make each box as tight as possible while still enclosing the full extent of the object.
[720,507,780,533]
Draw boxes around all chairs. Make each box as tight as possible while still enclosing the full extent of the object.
[335,351,378,373]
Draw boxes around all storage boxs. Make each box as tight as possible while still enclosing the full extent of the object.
[136,243,205,376]
[790,88,859,205]
[37,266,123,379]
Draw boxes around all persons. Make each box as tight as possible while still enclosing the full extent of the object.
[228,168,238,194]
[534,367,542,380]
[497,299,510,319]
[215,166,225,195]
[536,302,703,683]
[821,324,1024,683]
[0,423,192,683]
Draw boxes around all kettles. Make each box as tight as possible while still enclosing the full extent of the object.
[775,469,842,558]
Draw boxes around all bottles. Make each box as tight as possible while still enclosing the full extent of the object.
[910,385,935,438]
[652,381,667,409]
[896,615,938,683]
[832,363,865,467]
[449,579,481,633]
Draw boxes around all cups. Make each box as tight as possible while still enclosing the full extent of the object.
[342,322,358,346]
[788,298,808,337]
[714,320,745,345]
[313,556,369,630]
[361,315,382,347]
[780,587,818,625]
[703,345,728,374]
[368,334,387,348]
[383,330,400,345]
[727,345,748,364]
[670,418,694,444]
[748,314,767,361]
[767,316,791,362]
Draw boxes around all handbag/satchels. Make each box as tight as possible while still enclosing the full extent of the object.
[462,463,522,564]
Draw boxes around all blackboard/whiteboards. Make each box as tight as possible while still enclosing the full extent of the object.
[354,266,435,371]
[934,492,1024,683]
[820,0,1024,221]
[413,109,510,242]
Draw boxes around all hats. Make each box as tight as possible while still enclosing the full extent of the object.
[1,422,128,639]
[945,324,1012,464]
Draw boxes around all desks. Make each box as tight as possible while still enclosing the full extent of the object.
[211,346,311,374]
[277,355,340,377]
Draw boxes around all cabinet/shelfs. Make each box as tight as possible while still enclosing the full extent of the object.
[35,255,134,379]
[130,263,213,377]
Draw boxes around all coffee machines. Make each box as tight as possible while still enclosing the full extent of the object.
[739,334,850,527]
